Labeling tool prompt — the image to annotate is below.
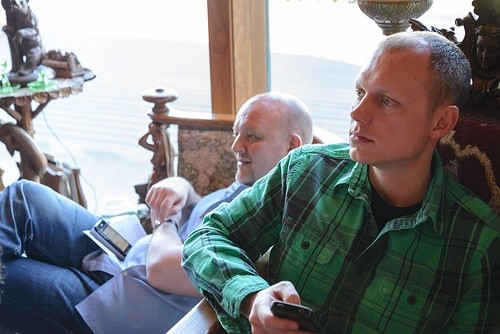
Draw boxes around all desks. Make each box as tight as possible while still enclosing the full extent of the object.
[0,56,96,138]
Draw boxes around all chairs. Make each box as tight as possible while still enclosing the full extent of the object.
[133,88,327,200]
[0,120,90,210]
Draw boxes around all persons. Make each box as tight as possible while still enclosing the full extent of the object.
[467,24,500,110]
[0,91,314,334]
[181,30,500,334]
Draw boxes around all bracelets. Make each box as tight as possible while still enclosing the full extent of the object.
[154,219,178,233]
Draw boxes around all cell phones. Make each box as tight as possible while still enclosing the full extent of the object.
[90,218,132,260]
[270,302,323,334]
